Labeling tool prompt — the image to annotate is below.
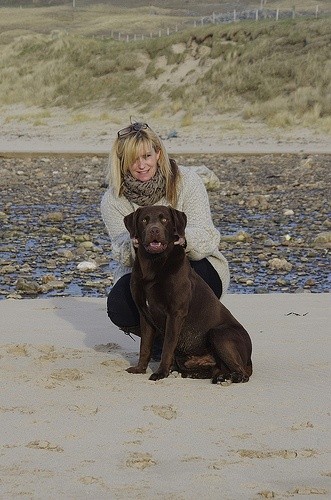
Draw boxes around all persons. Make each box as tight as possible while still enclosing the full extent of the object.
[101,120,230,362]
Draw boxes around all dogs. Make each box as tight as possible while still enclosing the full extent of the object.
[123,205,253,386]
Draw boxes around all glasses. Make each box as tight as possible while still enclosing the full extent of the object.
[116,121,154,142]
